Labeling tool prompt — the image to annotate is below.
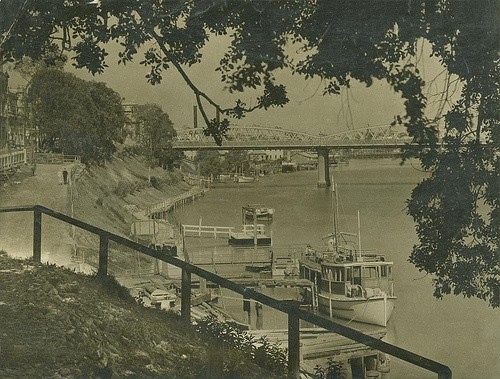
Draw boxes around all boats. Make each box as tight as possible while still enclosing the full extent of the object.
[297,176,397,327]
[235,175,255,183]
[243,204,275,224]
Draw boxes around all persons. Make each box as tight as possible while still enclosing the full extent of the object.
[63,167,68,184]
